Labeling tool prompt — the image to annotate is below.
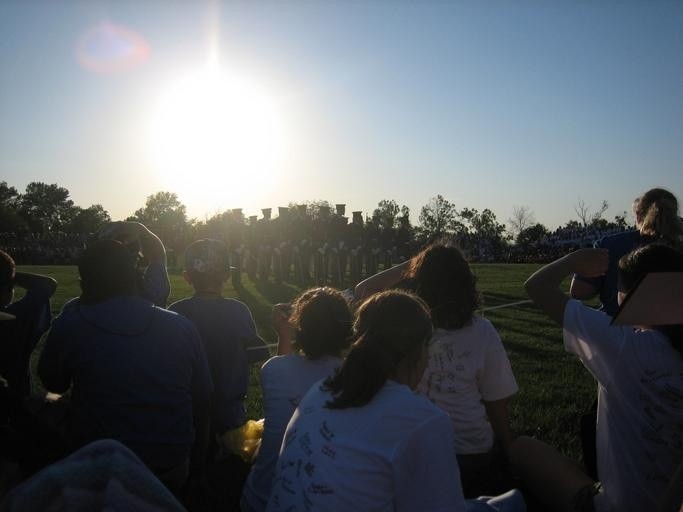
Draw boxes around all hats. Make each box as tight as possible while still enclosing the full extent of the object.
[319,204,393,227]
[184,239,236,275]
[232,204,307,223]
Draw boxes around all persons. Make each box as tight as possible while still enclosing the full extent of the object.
[500,186,683,510]
[0,222,524,511]
